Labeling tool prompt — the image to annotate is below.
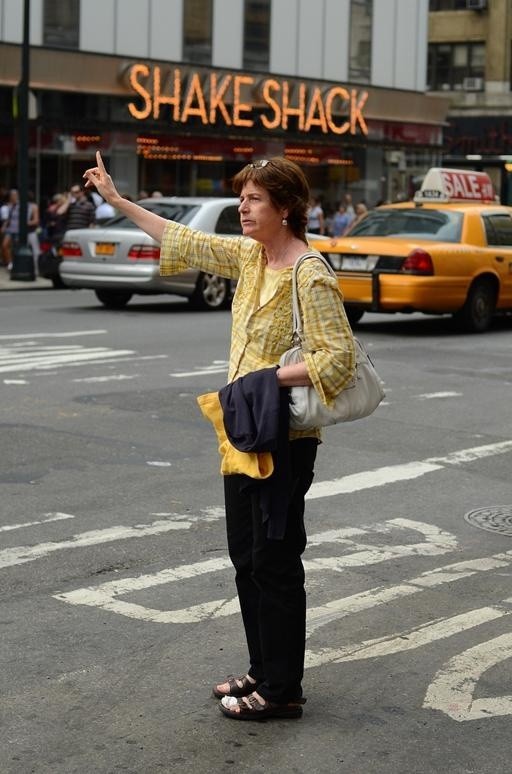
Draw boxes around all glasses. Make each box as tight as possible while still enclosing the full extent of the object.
[71,189,81,195]
[248,158,285,182]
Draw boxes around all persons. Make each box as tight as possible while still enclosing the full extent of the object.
[83,141,357,723]
[306,191,370,236]
[0,182,166,277]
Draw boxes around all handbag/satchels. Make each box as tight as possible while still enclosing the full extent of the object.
[275,253,387,429]
[38,248,58,279]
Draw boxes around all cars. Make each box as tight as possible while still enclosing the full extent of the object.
[304,167,512,335]
[58,196,332,311]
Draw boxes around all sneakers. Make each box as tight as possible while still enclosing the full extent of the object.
[7,262,13,270]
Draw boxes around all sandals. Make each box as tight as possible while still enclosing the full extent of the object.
[220,688,304,722]
[212,671,260,697]
